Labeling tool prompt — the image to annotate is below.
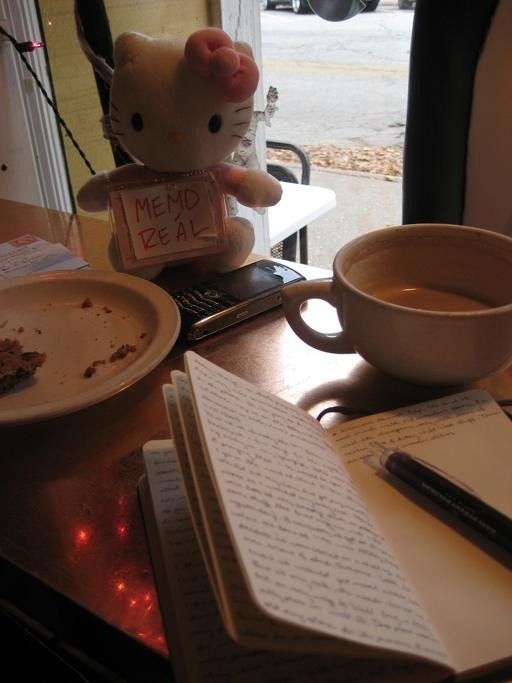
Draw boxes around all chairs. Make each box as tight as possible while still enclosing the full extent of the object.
[266,139,308,267]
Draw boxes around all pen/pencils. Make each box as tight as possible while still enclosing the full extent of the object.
[378,448,512,571]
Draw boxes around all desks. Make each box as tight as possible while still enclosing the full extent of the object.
[247,181,336,265]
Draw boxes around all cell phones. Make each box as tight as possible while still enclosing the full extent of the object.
[169,255,306,343]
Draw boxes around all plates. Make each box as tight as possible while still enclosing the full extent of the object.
[0,266,183,427]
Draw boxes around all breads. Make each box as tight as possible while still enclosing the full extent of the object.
[0,339,47,395]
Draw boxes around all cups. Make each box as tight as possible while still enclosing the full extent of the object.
[279,220,512,385]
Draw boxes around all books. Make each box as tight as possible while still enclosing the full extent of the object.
[138,347,512,680]
